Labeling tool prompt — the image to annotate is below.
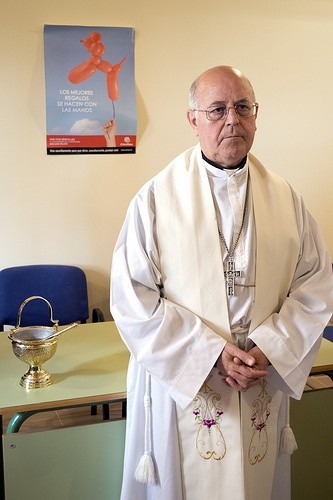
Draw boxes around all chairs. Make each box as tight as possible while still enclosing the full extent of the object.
[0,265,111,422]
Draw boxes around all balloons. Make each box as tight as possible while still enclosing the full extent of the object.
[66,29,128,100]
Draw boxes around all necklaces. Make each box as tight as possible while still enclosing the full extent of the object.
[211,170,249,296]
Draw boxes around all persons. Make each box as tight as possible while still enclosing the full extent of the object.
[108,64,333,500]
[102,117,119,146]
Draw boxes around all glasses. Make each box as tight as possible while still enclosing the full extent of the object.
[192,103,257,122]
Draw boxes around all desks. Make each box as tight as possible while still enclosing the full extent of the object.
[0,316,333,500]
